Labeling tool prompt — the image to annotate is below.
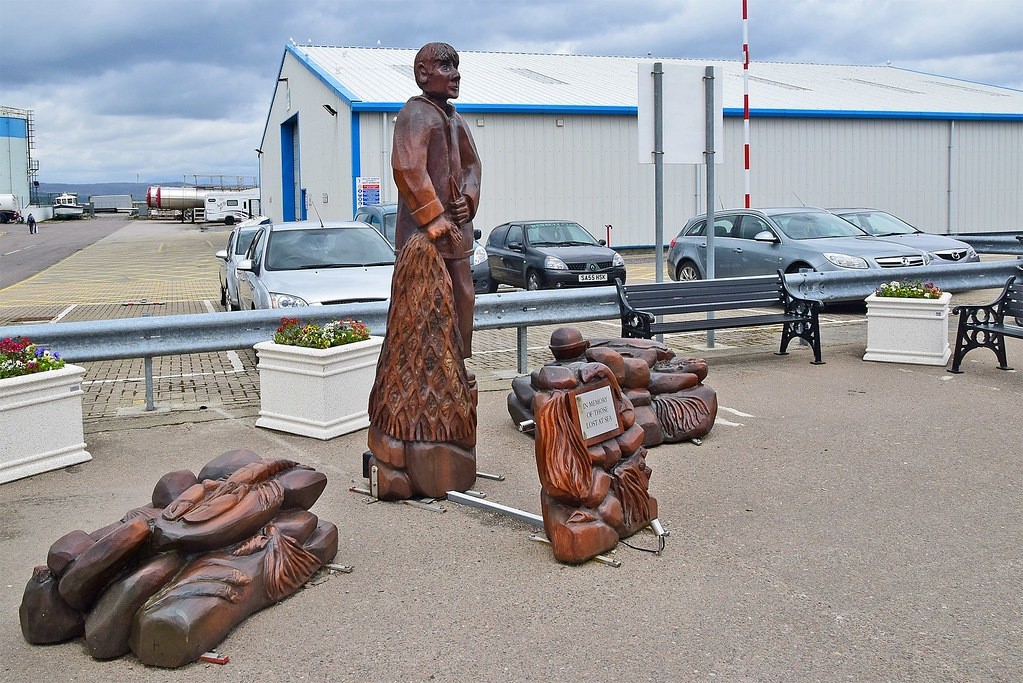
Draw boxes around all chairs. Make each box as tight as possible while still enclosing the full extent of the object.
[540,227,558,240]
[514,233,530,242]
[786,217,816,239]
[709,225,728,236]
[742,220,765,238]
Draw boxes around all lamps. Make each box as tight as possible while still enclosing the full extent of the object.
[255,149,264,155]
[323,104,336,115]
[278,77,288,81]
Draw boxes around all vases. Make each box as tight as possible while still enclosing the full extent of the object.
[0,364,94,486]
[862,291,954,368]
[254,336,388,441]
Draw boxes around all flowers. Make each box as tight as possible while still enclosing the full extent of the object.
[876,279,940,298]
[273,314,370,349]
[0,337,69,380]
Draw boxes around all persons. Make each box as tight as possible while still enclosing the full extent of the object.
[27,213,35,234]
[389,42,484,391]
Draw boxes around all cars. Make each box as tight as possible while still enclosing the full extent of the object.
[819,205,980,264]
[663,205,933,294]
[235,219,397,312]
[215,224,268,313]
[483,218,627,293]
[352,201,492,295]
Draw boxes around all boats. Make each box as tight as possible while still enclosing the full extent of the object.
[52,194,83,217]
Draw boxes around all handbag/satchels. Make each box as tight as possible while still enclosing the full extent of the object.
[33,224,38,234]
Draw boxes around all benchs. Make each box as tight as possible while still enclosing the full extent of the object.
[613,268,827,365]
[948,274,1023,376]
